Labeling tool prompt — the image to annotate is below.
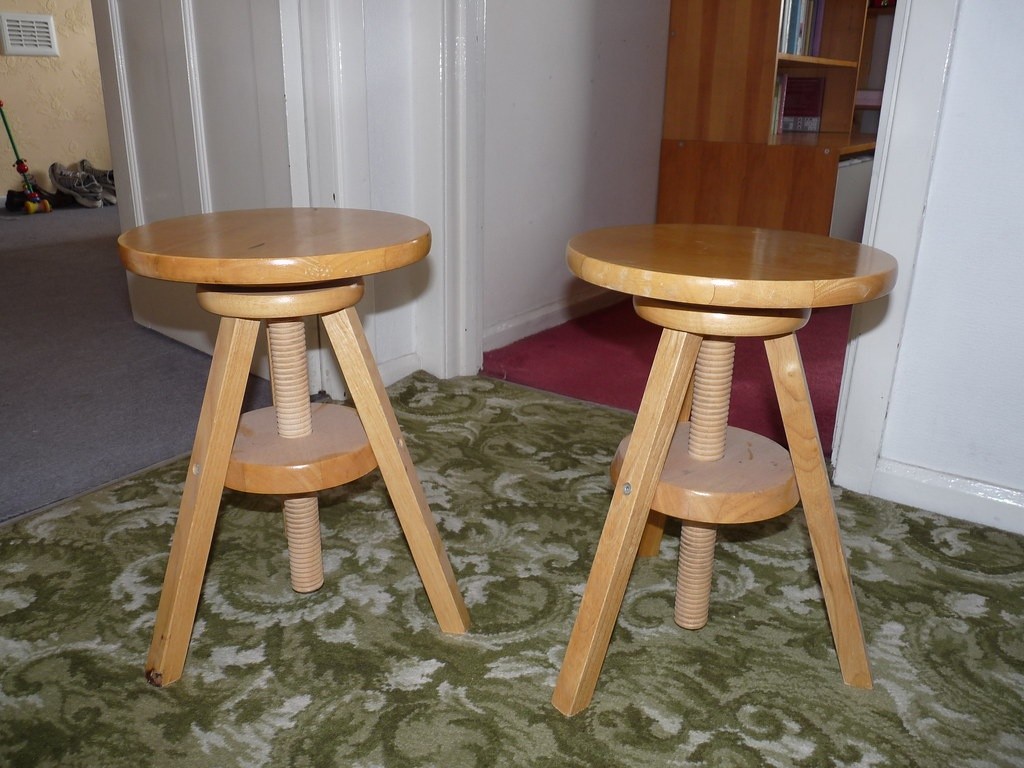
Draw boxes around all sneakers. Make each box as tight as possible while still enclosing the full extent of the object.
[48,162,103,208]
[77,159,118,205]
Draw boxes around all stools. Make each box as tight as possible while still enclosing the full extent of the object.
[550,224,899,716]
[116,207,470,686]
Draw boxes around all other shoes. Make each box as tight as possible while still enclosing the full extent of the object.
[5,181,77,211]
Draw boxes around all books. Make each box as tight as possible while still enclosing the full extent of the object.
[778,0,823,56]
[771,69,824,137]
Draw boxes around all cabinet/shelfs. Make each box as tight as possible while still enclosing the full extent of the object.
[658,0,897,246]
